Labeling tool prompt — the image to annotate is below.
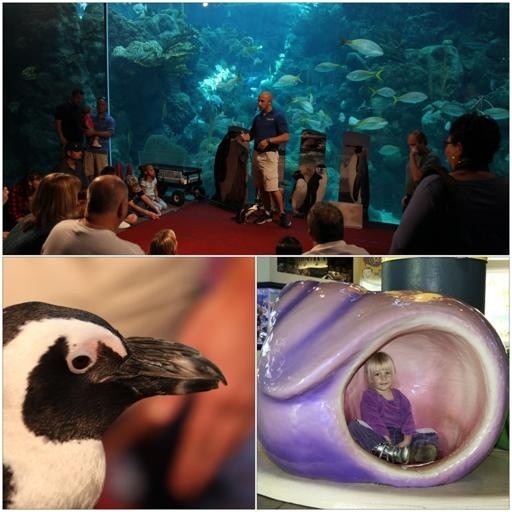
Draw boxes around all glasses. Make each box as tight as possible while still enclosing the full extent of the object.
[442,139,453,146]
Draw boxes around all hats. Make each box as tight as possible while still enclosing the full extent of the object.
[64,145,84,152]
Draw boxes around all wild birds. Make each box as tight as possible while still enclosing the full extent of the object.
[2,299,229,510]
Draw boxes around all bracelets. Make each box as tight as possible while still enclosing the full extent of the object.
[265,138,272,144]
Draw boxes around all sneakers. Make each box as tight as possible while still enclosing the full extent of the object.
[254,210,273,224]
[279,213,291,227]
[92,139,100,147]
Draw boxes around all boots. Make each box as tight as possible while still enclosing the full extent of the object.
[375,440,409,462]
[409,444,437,463]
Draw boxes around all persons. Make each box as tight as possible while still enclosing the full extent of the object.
[348,351,440,465]
[400,129,441,212]
[303,201,370,255]
[3,87,178,254]
[276,235,303,255]
[237,91,292,228]
[391,111,509,253]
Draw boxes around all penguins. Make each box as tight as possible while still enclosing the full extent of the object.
[304,164,328,210]
[289,169,307,214]
[344,143,370,205]
